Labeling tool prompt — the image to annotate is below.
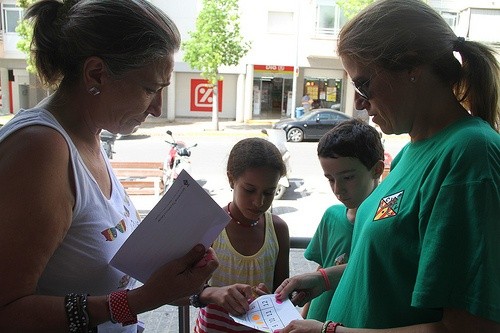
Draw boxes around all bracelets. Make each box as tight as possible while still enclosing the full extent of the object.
[321,320,332,333]
[65,292,90,333]
[318,267,332,290]
[105,288,138,326]
[326,322,340,333]
[188,283,209,308]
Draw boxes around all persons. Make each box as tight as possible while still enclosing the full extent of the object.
[300,118,385,322]
[273,0,500,333]
[0,0,219,333]
[166,137,290,333]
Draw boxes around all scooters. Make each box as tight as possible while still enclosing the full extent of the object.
[160,129,199,197]
[99,132,117,159]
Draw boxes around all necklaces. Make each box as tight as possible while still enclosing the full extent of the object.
[227,202,260,228]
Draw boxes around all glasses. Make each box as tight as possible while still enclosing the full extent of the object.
[350,69,384,102]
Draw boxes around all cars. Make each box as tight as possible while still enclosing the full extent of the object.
[271,107,354,143]
